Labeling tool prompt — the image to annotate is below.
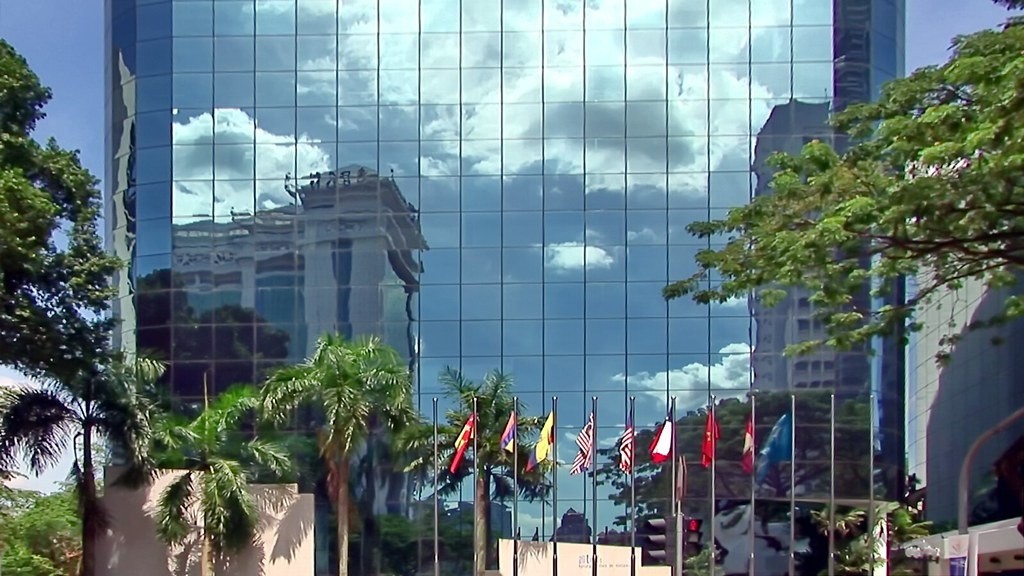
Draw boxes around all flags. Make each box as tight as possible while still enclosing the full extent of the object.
[616,413,633,474]
[569,411,593,475]
[700,410,721,468]
[501,412,513,453]
[525,411,553,472]
[754,412,791,485]
[451,413,476,474]
[648,408,673,465]
[740,415,756,472]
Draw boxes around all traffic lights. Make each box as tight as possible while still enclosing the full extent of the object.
[682,516,703,559]
[641,516,676,567]
